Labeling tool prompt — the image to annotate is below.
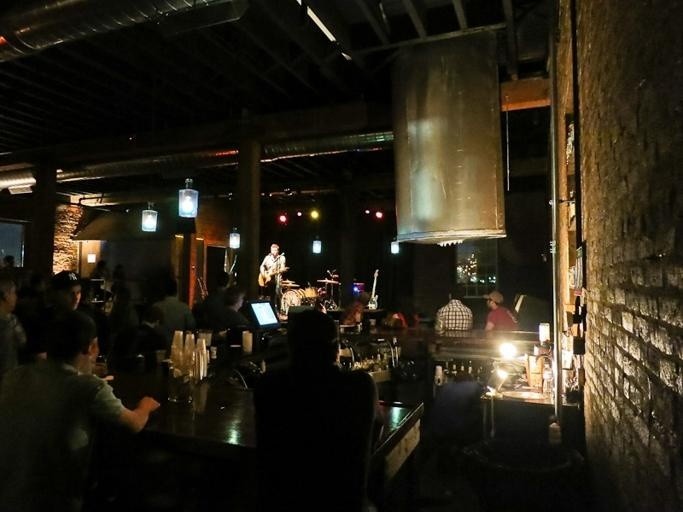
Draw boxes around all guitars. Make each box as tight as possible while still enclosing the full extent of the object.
[366,269,380,310]
[258,267,289,287]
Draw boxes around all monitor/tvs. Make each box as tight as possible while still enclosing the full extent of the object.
[248,300,281,328]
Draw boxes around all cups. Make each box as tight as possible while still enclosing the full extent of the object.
[242,331,253,354]
[171,330,217,380]
[94,355,107,375]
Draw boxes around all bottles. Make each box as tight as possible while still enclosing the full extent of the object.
[549,418,562,447]
[542,362,554,400]
[434,359,485,386]
[343,352,391,373]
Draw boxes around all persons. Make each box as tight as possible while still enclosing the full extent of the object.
[431,371,484,446]
[483,291,522,331]
[434,286,474,337]
[259,244,286,310]
[0,311,161,512]
[253,310,389,512]
[0,260,255,372]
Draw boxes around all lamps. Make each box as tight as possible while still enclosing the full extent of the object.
[141,175,198,233]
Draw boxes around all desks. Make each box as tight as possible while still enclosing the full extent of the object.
[104,370,423,483]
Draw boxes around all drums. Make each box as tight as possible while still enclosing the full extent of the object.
[281,290,308,315]
[304,287,318,298]
[317,287,327,299]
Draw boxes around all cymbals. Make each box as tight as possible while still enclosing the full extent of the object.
[317,279,338,284]
[280,283,300,287]
[281,280,295,284]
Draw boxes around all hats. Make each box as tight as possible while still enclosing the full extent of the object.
[449,285,466,298]
[483,291,503,303]
[51,270,85,289]
